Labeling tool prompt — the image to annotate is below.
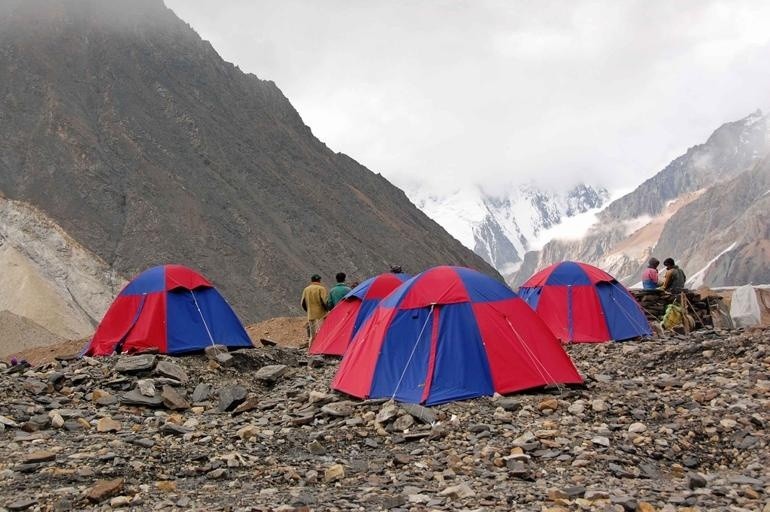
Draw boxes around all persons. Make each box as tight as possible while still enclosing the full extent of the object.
[642,257,664,289]
[301,274,328,349]
[327,274,353,309]
[663,257,686,292]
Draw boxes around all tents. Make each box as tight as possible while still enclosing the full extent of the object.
[517,261,653,344]
[329,265,584,406]
[308,272,414,358]
[82,264,255,356]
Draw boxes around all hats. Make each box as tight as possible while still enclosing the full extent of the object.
[311,274,321,280]
[649,257,659,265]
[664,258,674,266]
